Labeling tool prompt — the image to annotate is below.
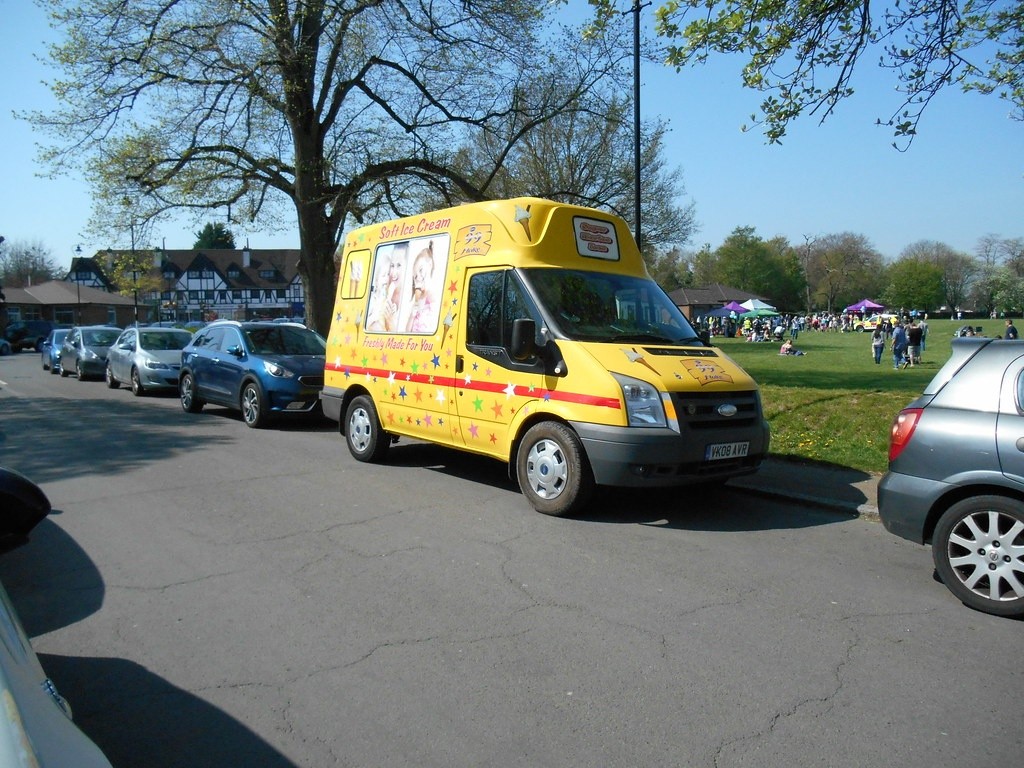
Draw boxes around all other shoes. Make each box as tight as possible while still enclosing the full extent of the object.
[893,367,899,370]
[903,361,909,369]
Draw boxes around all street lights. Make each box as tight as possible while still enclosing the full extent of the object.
[72,243,84,327]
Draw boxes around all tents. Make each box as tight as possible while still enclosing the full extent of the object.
[705,299,779,320]
[847,300,885,315]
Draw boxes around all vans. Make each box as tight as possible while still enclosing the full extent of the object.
[319,196,768,518]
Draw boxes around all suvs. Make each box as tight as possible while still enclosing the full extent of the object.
[178,320,328,429]
[5,318,63,353]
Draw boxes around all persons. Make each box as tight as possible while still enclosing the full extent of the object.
[369,243,411,332]
[964,331,1003,340]
[406,241,437,334]
[690,307,928,371]
[1005,320,1018,342]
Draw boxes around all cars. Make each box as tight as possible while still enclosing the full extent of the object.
[854,315,900,333]
[40,329,101,374]
[59,327,124,381]
[126,315,307,331]
[106,328,195,396]
[0,339,11,356]
[875,337,1024,618]
